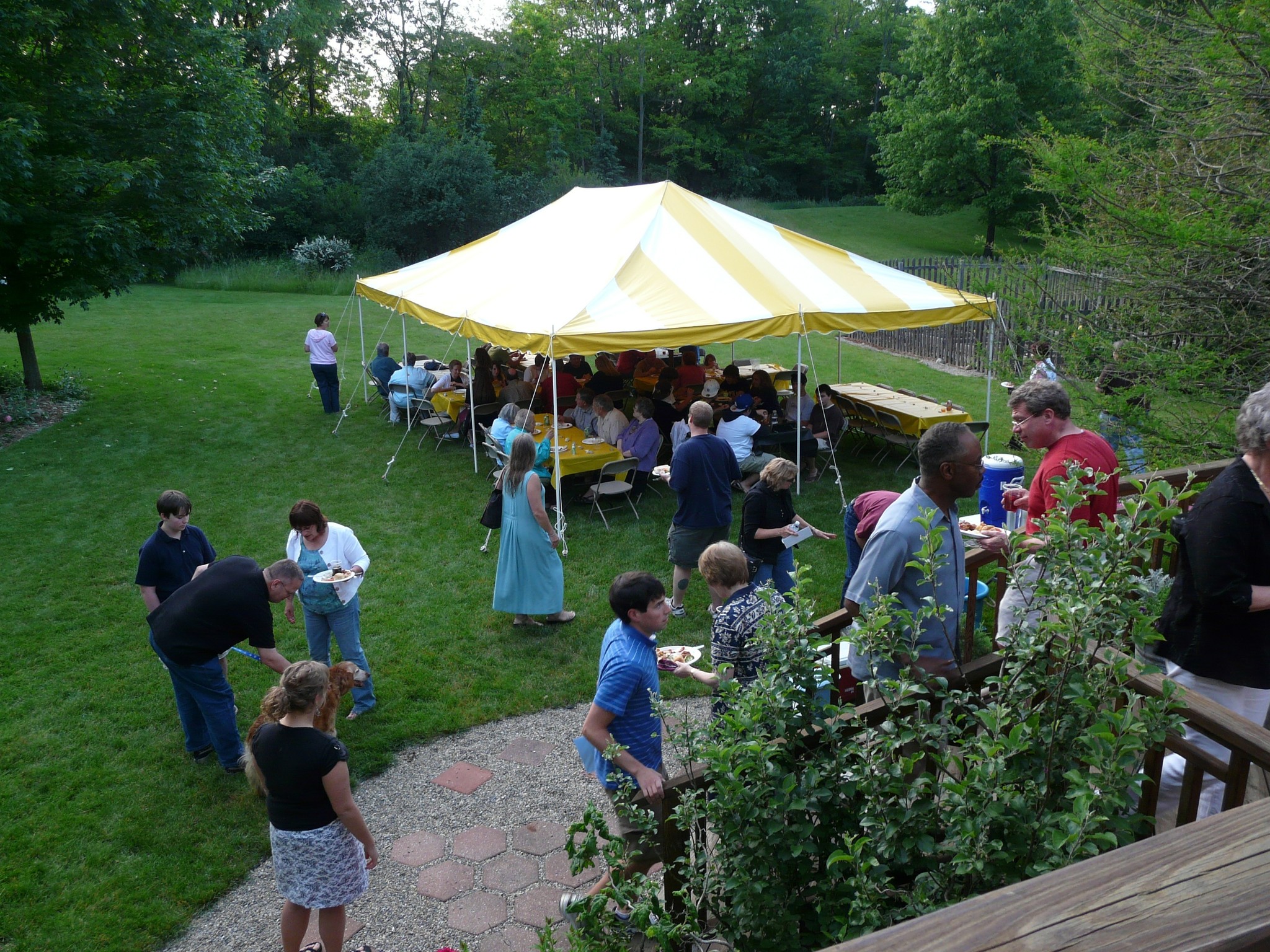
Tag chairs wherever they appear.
[359,345,808,527]
[813,382,990,482]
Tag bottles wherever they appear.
[782,521,800,544]
[764,414,769,424]
[771,410,778,428]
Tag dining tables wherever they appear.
[430,377,586,422]
[633,362,790,393]
[510,413,629,490]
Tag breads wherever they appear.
[976,523,1001,531]
[673,654,685,663]
[333,572,345,581]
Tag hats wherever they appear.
[702,379,719,398]
[730,394,753,412]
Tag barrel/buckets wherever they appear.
[963,577,996,631]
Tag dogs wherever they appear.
[236,659,373,800]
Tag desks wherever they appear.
[398,358,474,382]
[814,381,973,439]
[652,347,682,366]
[506,353,568,372]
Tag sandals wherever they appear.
[348,945,376,952]
[347,712,358,721]
[733,482,747,493]
[300,942,322,952]
[513,614,544,627]
[546,611,576,622]
[575,496,594,503]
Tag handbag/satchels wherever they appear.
[740,549,763,585]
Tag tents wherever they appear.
[355,181,996,538]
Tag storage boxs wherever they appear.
[818,642,859,704]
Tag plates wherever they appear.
[454,389,466,393]
[715,396,732,401]
[1001,382,1014,387]
[960,527,1011,539]
[576,379,585,382]
[706,368,718,372]
[462,395,466,398]
[532,429,541,435]
[705,373,720,377]
[718,370,723,375]
[551,423,572,429]
[655,646,702,668]
[313,569,355,583]
[550,446,567,452]
[652,466,670,476]
[651,374,659,378]
[582,438,605,444]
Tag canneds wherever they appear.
[543,415,550,426]
[584,427,591,438]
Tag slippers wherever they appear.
[805,476,817,483]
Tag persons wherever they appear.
[1002,341,1057,451]
[1095,340,1153,474]
[977,379,1120,650]
[304,312,346,414]
[673,541,793,748]
[559,571,671,940]
[370,344,903,613]
[134,490,239,714]
[145,558,305,774]
[251,660,379,952]
[844,421,986,796]
[284,499,376,721]
[1094,380,1270,822]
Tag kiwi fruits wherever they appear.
[684,655,694,662]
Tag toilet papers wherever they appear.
[756,409,768,416]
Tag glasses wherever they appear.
[785,478,795,483]
[1012,412,1036,428]
[947,459,984,470]
[281,582,294,600]
[320,312,325,318]
[295,523,312,533]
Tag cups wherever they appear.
[941,402,947,412]
[331,560,342,576]
[713,363,717,368]
[450,385,456,392]
[662,348,666,354]
[585,375,591,382]
[1003,484,1023,511]
[540,374,547,386]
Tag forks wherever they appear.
[652,477,661,481]
[963,538,980,547]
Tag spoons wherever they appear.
[970,544,982,548]
[665,661,677,667]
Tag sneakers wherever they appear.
[559,893,589,932]
[609,906,659,935]
[194,743,215,761]
[665,596,685,618]
[225,764,246,774]
[708,604,718,617]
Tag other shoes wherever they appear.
[469,444,474,448]
[443,433,459,438]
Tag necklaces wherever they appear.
[1250,468,1270,494]
